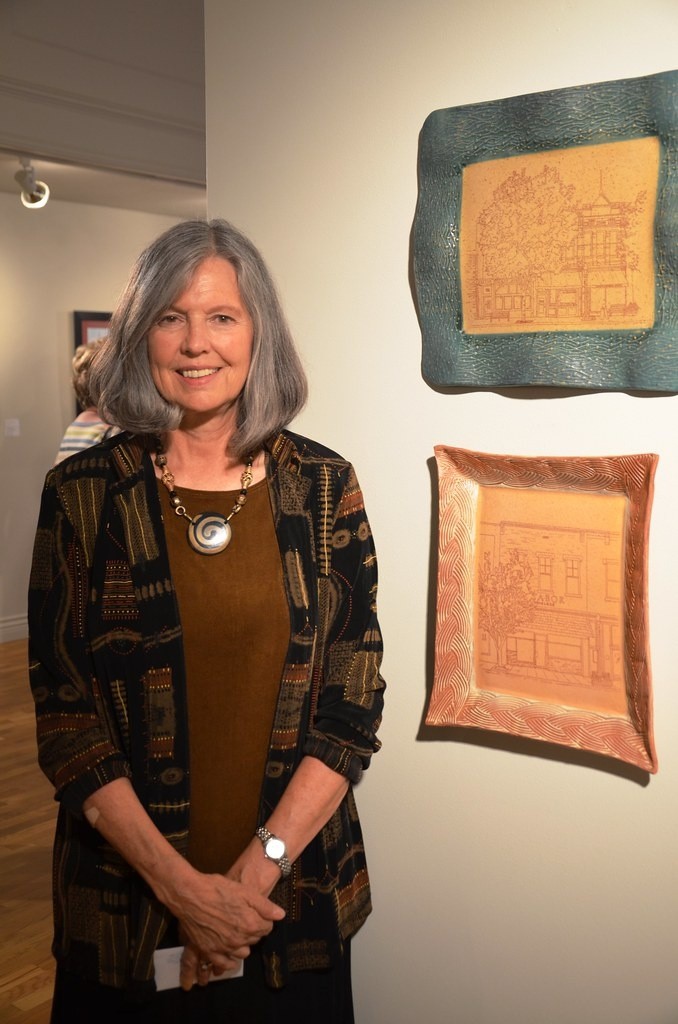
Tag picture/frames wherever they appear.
[73,310,112,416]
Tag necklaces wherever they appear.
[155,450,251,555]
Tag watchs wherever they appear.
[255,826,291,878]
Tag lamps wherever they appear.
[16,154,50,209]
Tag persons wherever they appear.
[53,335,120,466]
[28,206,387,1024]
